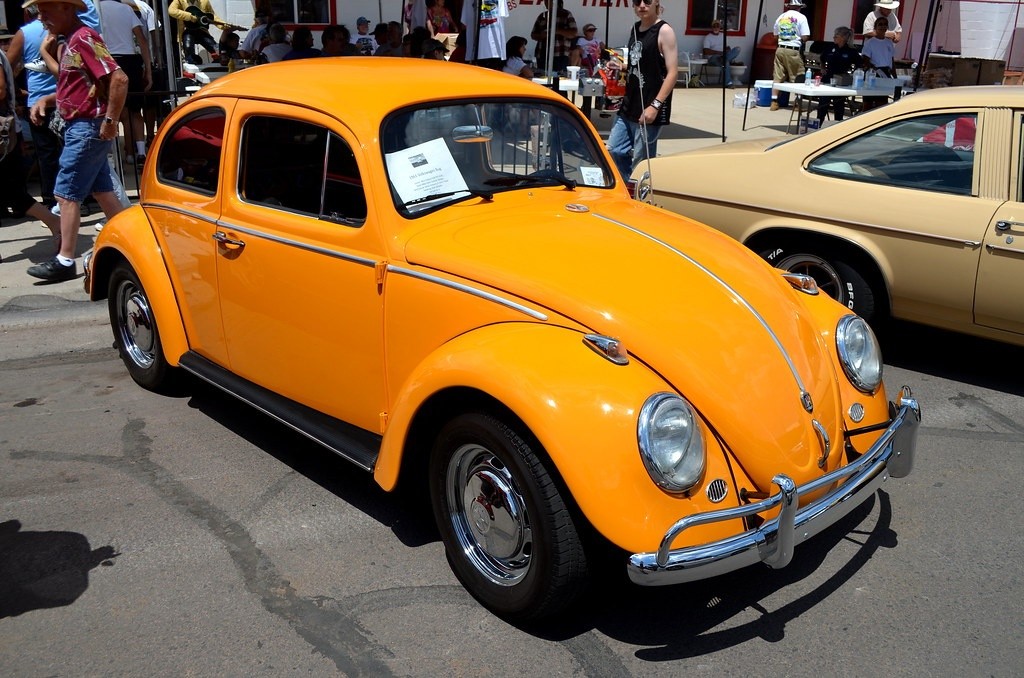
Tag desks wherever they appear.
[682,58,708,87]
[184,63,250,84]
[524,67,578,105]
[774,82,931,134]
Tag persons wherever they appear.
[605,0,679,182]
[530,0,578,98]
[20,0,129,281]
[96,1,153,169]
[216,7,320,60]
[6,0,58,211]
[370,21,449,61]
[702,20,741,90]
[119,0,162,163]
[348,16,378,55]
[122,2,156,162]
[317,25,361,55]
[503,36,533,79]
[569,25,610,72]
[817,0,907,128]
[404,0,459,37]
[769,0,809,111]
[167,0,231,64]
[0,48,62,263]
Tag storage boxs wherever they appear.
[732,97,756,108]
[754,80,790,107]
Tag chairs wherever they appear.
[699,52,724,86]
[660,48,691,89]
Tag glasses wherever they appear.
[713,25,720,28]
[632,0,652,6]
[588,29,596,32]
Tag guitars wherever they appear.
[182,5,250,32]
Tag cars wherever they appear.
[628,85,1024,347]
[84,56,922,626]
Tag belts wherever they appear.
[777,45,799,50]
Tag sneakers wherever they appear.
[23,57,51,73]
[27,256,76,281]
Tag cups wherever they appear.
[690,53,695,60]
[830,78,837,87]
[699,54,703,59]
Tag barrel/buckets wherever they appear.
[566,66,580,80]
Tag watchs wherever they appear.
[104,117,118,126]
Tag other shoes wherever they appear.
[770,102,779,111]
[54,233,61,254]
[717,56,724,62]
[795,104,807,111]
[94,217,109,231]
[727,84,734,88]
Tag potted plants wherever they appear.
[729,60,748,85]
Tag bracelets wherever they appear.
[619,63,622,71]
[650,99,663,110]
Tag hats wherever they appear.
[21,0,88,12]
[874,0,900,9]
[357,17,371,24]
[255,8,268,18]
[369,23,388,35]
[422,38,449,54]
[585,26,597,31]
[711,20,720,25]
[784,0,807,8]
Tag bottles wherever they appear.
[852,68,865,89]
[228,58,235,74]
[864,66,876,89]
[805,68,812,86]
[815,76,820,87]
